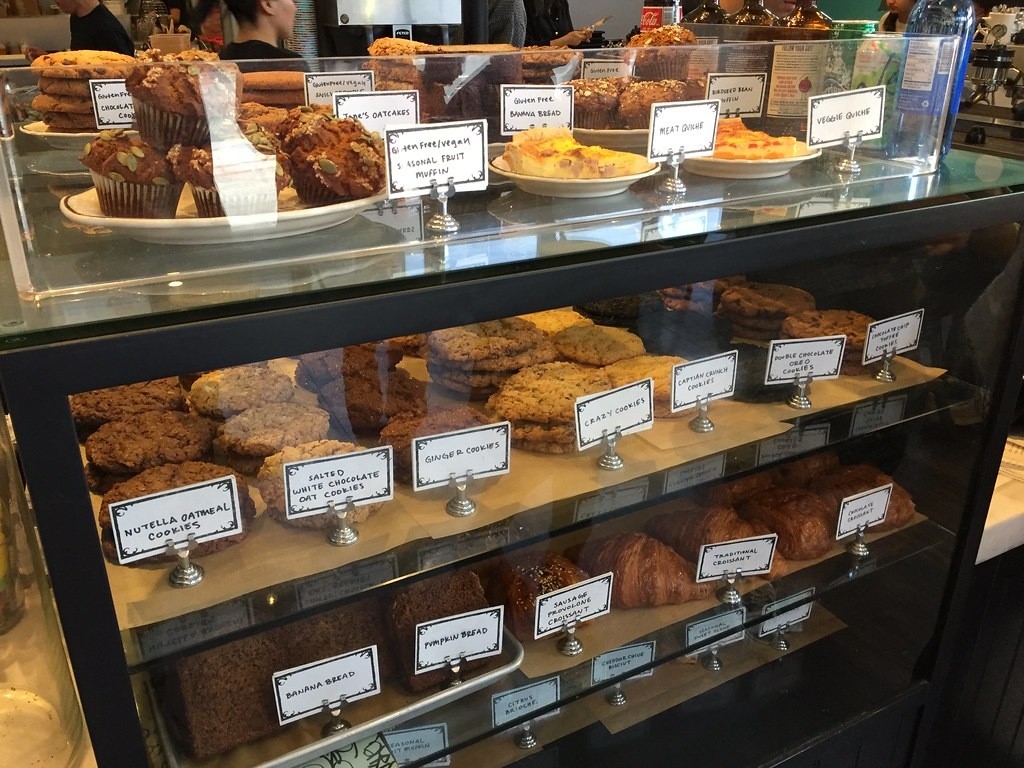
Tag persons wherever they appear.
[23,0,993,74]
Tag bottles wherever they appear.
[640,0,680,33]
[0,483,27,637]
[885,1,975,164]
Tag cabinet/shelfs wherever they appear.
[0,123,1024,768]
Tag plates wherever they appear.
[487,152,663,199]
[146,609,528,768]
[681,141,827,179]
[19,121,113,149]
[572,126,653,152]
[56,183,392,249]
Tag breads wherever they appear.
[30,22,916,760]
